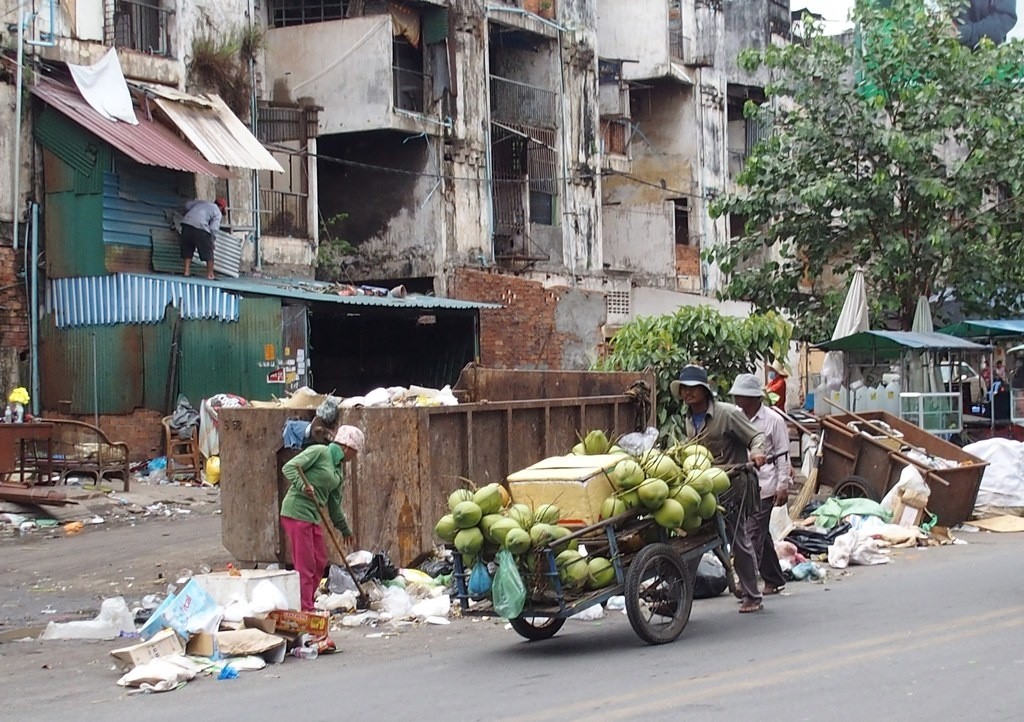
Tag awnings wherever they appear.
[808,329,995,429]
[0,49,287,180]
[932,319,1023,427]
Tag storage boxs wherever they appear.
[847,419,903,451]
[507,454,630,528]
[195,568,301,623]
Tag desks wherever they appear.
[0,423,55,485]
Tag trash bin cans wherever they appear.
[944,382,973,413]
[214,361,658,571]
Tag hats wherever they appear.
[670,364,717,399]
[334,425,365,453]
[727,373,766,397]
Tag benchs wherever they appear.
[20,418,129,492]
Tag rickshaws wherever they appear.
[444,449,788,644]
[771,406,991,528]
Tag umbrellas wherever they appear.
[903,296,949,435]
[819,265,871,412]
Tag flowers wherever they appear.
[10,387,30,404]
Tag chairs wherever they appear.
[162,415,202,482]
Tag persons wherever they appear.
[180,197,226,283]
[279,424,364,614]
[983,359,1006,390]
[767,360,786,413]
[725,373,795,596]
[671,365,766,613]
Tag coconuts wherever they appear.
[571,429,731,532]
[435,483,615,590]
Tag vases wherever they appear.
[14,403,23,423]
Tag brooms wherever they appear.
[788,428,825,523]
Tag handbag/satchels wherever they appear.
[468,556,494,602]
[493,551,526,619]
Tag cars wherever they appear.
[939,362,988,415]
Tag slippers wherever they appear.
[761,585,785,595]
[650,601,677,617]
[738,603,764,613]
